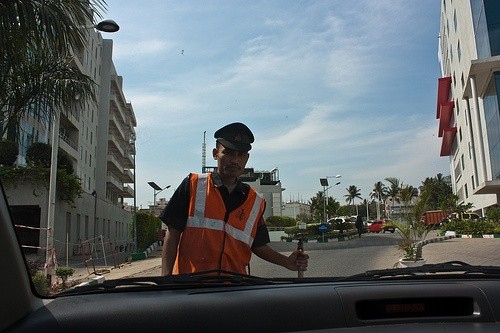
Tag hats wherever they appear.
[214,123,254,151]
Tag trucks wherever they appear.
[421,209,481,225]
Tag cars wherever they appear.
[328,215,395,234]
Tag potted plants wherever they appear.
[381,177,448,269]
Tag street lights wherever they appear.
[148,182,171,206]
[46,19,120,289]
[320,177,341,225]
